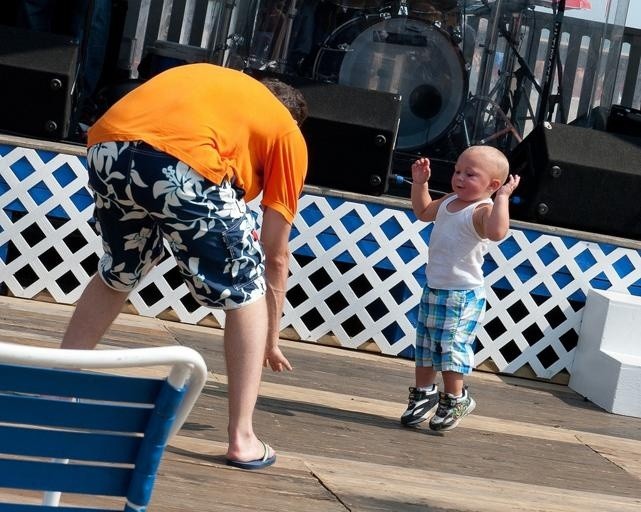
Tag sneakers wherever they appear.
[399,383,441,427]
[428,383,477,433]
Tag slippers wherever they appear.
[223,435,277,471]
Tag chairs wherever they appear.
[0,343,207,512]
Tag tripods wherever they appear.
[422,0,525,163]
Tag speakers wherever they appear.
[0,25,80,139]
[490,121,641,241]
[234,65,402,196]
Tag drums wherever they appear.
[310,13,468,150]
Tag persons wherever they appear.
[402,144,521,435]
[40,62,308,468]
